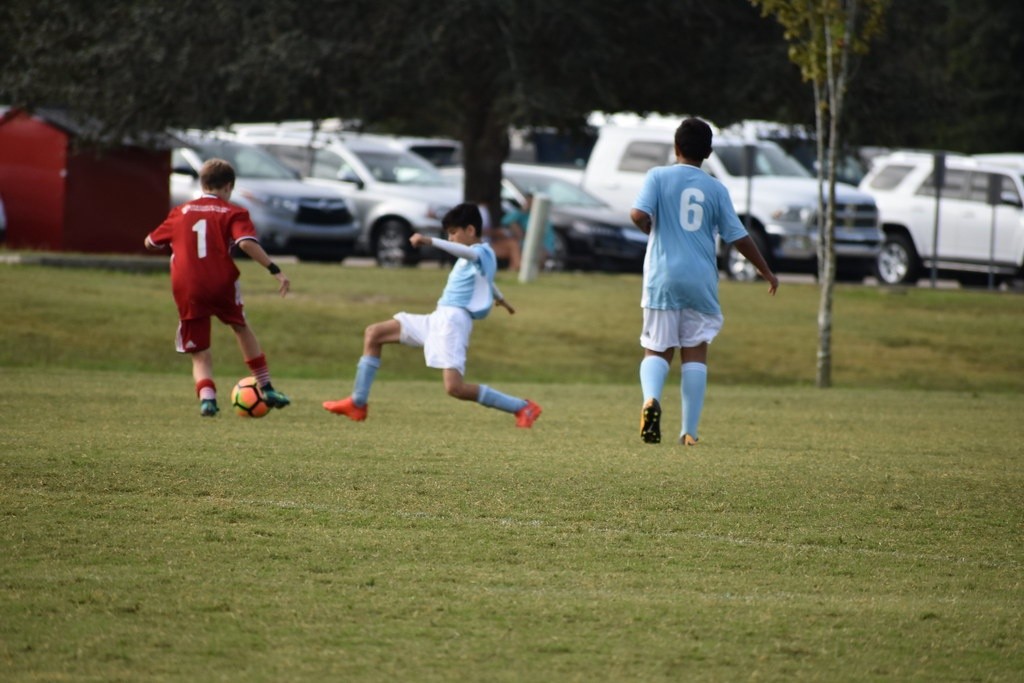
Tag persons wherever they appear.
[321,202,543,430]
[629,118,780,447]
[143,157,293,417]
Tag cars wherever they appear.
[0,80,1024,297]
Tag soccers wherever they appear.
[231,376,272,418]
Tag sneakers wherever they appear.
[262,391,290,409]
[322,397,367,422]
[202,398,216,416]
[515,399,541,428]
[639,399,662,444]
[681,434,702,446]
